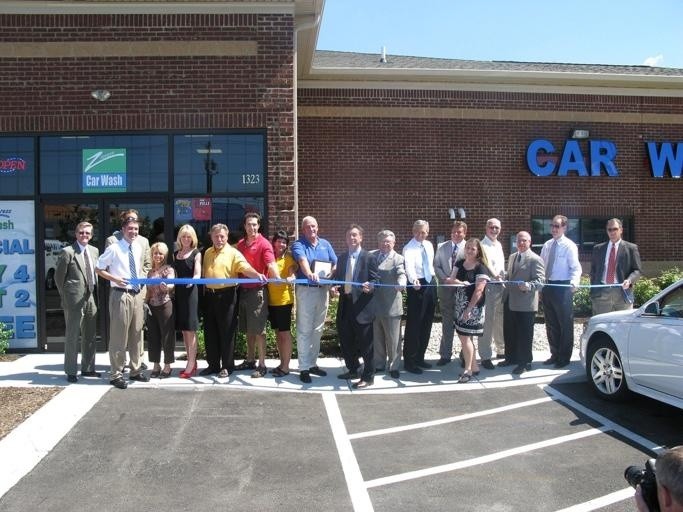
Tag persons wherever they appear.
[402,220,434,375]
[479,218,506,370]
[329,223,378,389]
[95,216,149,389]
[171,225,201,379]
[235,212,282,379]
[367,230,407,378]
[635,446,683,512]
[145,242,175,379]
[290,216,337,383]
[434,220,468,368]
[104,209,152,373]
[54,222,100,383]
[266,230,299,377]
[498,231,544,370]
[199,224,267,377]
[588,218,642,317]
[537,215,582,368]
[449,238,491,383]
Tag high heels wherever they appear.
[150,368,161,378]
[159,368,172,379]
[180,364,197,378]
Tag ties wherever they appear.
[345,256,353,294]
[606,243,615,285]
[546,242,557,281]
[378,254,385,264]
[129,244,139,293]
[453,244,458,268]
[84,249,94,293]
[421,243,432,284]
[518,254,522,265]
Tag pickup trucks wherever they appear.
[44,239,64,289]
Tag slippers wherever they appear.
[458,373,472,383]
[272,370,289,377]
[269,367,281,373]
[472,370,481,375]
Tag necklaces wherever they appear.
[177,247,189,258]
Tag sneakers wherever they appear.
[234,361,256,370]
[251,366,267,378]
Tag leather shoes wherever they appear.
[309,367,327,376]
[389,370,400,378]
[130,373,150,382]
[199,367,220,375]
[82,371,100,378]
[554,359,571,368]
[497,359,514,365]
[436,358,451,365]
[544,357,559,365]
[68,374,78,383]
[513,363,532,374]
[407,366,423,374]
[300,372,311,383]
[481,359,494,369]
[418,362,431,368]
[110,378,128,390]
[338,372,362,379]
[220,366,233,377]
[353,380,374,388]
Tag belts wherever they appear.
[546,280,570,284]
[299,283,328,287]
[205,286,236,294]
[114,284,144,293]
[603,286,620,288]
[345,294,351,299]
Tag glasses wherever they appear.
[550,223,563,228]
[607,227,620,232]
[125,217,141,222]
[78,231,91,235]
[379,240,393,244]
[487,226,500,229]
[516,239,528,242]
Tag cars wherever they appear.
[578,278,682,418]
